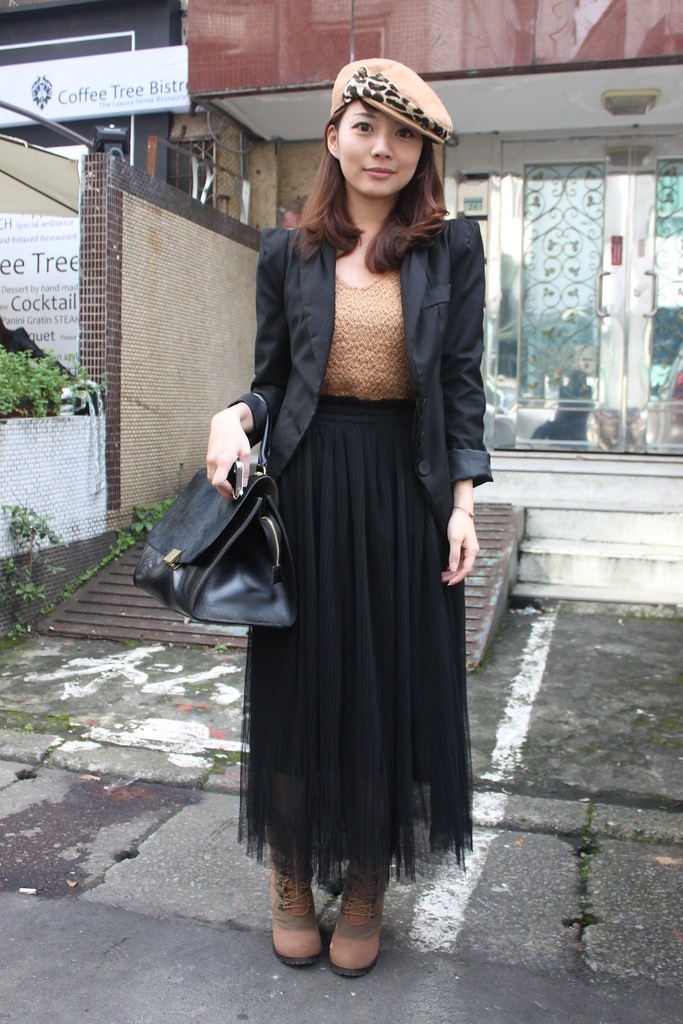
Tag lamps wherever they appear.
[607,146,653,168]
[601,88,661,117]
[92,124,130,156]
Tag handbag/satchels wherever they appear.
[132,390,298,627]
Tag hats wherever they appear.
[330,58,453,144]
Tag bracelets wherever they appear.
[453,505,474,517]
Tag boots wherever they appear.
[268,768,323,965]
[328,779,393,976]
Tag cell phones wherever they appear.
[228,462,245,497]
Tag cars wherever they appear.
[648,342,683,451]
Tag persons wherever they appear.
[205,59,523,975]
